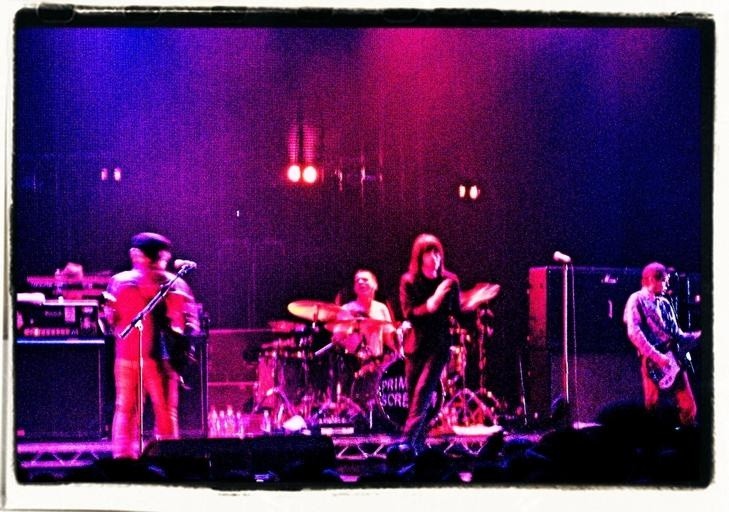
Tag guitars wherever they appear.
[647,326,704,391]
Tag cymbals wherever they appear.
[287,299,349,322]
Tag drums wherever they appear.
[352,359,444,429]
[254,350,327,410]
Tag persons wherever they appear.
[102,233,201,458]
[623,263,698,424]
[330,270,395,373]
[400,234,478,451]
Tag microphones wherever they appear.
[174,258,198,270]
[553,251,571,262]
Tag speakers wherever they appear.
[144,441,236,482]
[249,434,339,484]
